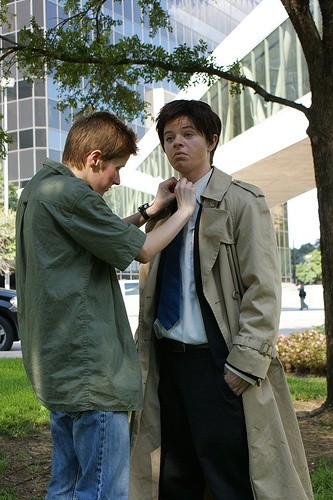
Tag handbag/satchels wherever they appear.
[299,289,306,297]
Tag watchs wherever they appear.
[137,203,151,221]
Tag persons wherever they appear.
[15,111,197,500]
[299,283,308,310]
[128,100,314,500]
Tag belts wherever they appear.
[156,336,210,353]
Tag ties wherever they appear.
[155,198,184,331]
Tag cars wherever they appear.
[0,288,21,350]
